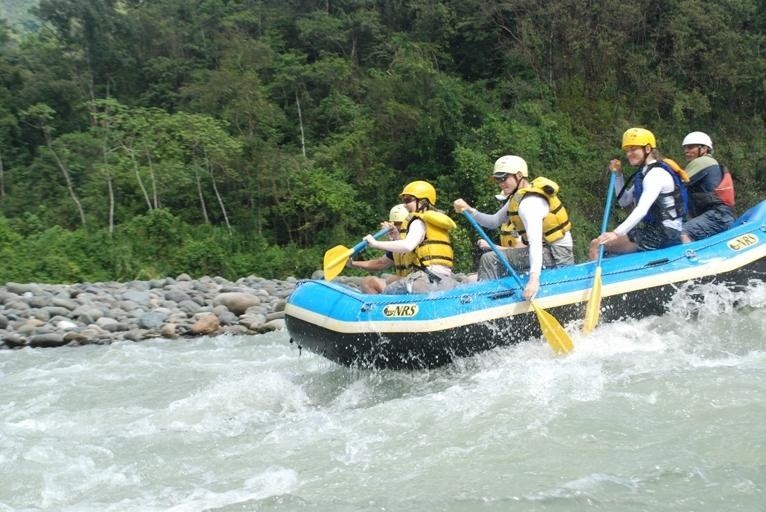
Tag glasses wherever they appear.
[495,177,507,183]
[403,196,416,203]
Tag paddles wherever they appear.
[583,160,618,334]
[322,227,396,282]
[460,208,574,355]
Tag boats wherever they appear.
[285,200,766,368]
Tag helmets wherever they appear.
[400,180,436,206]
[621,128,656,149]
[388,203,409,221]
[494,155,529,177]
[683,132,713,151]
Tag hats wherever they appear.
[490,172,506,178]
[494,194,506,201]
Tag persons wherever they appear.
[665,132,734,246]
[588,128,684,261]
[453,154,573,279]
[345,179,455,295]
